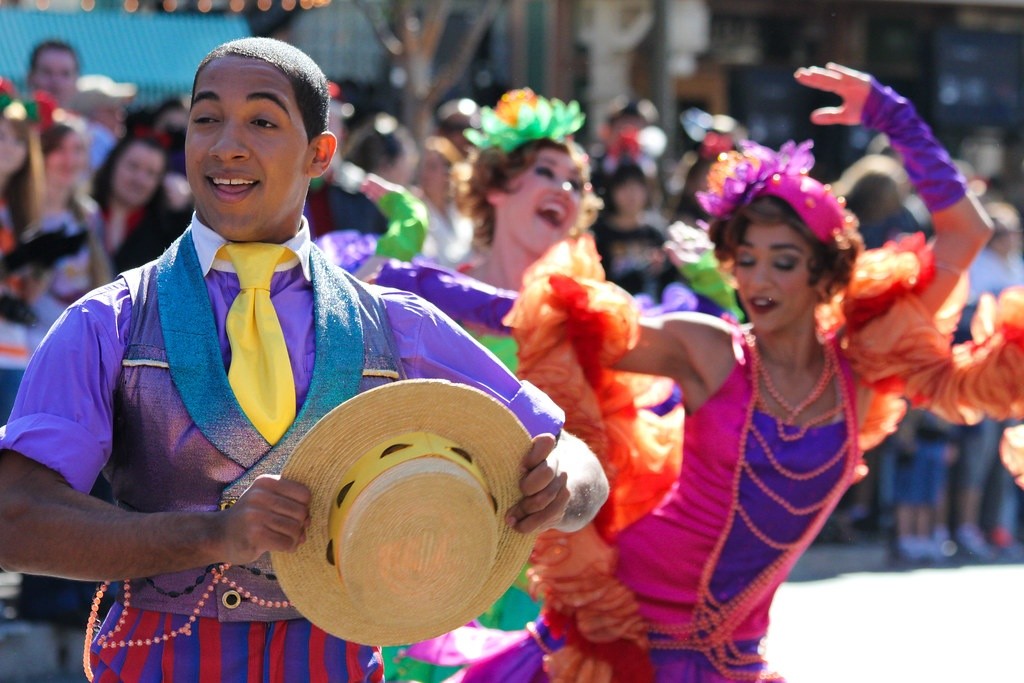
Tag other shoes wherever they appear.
[903,523,1024,562]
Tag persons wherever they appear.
[383,88,593,683]
[0,40,1024,567]
[0,36,610,683]
[369,62,996,683]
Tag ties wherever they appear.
[217,242,301,449]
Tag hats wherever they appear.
[69,75,136,112]
[270,378,547,647]
